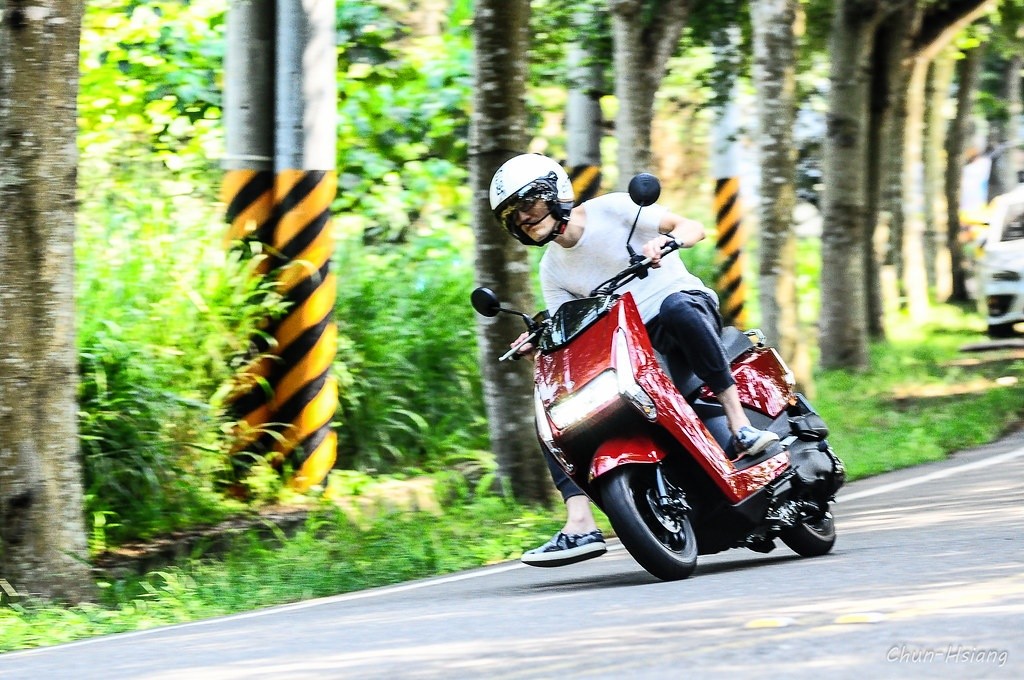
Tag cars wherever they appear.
[983,190,1023,337]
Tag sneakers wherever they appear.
[726,426,779,462]
[521,529,607,567]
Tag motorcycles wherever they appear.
[473,173,848,583]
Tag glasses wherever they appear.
[505,190,539,225]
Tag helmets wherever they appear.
[489,153,573,247]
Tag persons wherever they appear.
[489,152,780,568]
